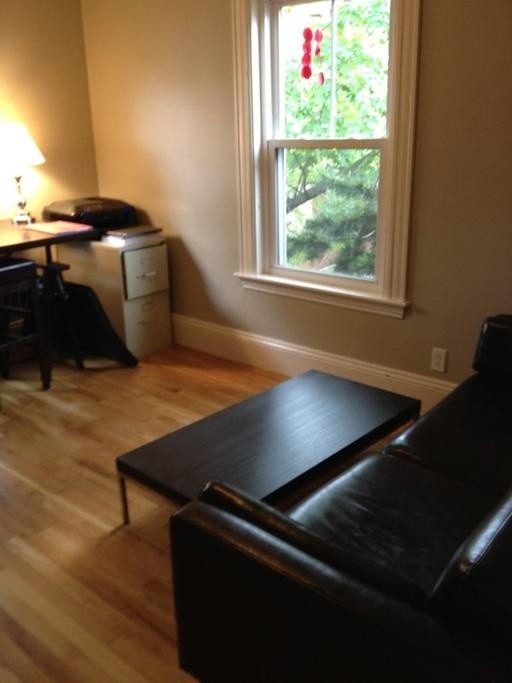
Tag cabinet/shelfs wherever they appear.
[57,235,174,359]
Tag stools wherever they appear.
[1,255,57,391]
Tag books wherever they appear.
[20,219,95,238]
[100,225,167,248]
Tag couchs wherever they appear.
[168,307,510,681]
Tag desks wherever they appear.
[0,216,97,372]
